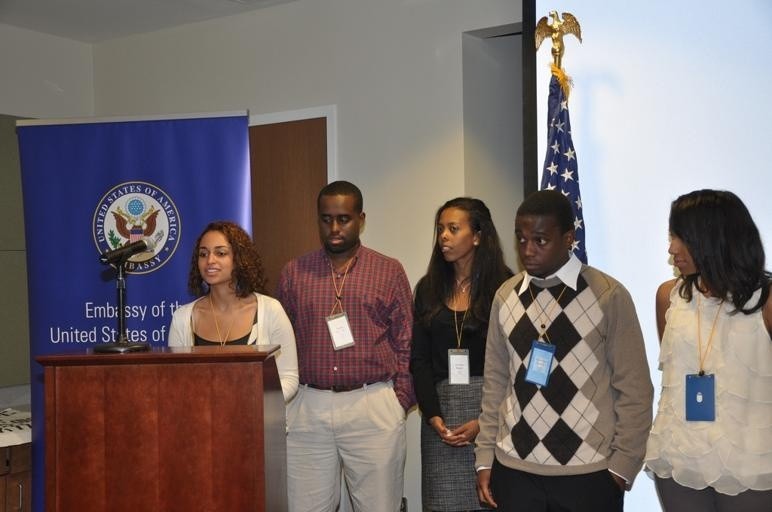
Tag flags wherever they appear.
[538,67,589,267]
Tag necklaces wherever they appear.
[454,278,472,293]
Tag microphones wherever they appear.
[98,237,153,265]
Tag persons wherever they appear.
[166,219,301,404]
[639,188,770,511]
[279,181,418,510]
[408,195,517,510]
[472,190,653,512]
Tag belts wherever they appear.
[300,381,378,392]
